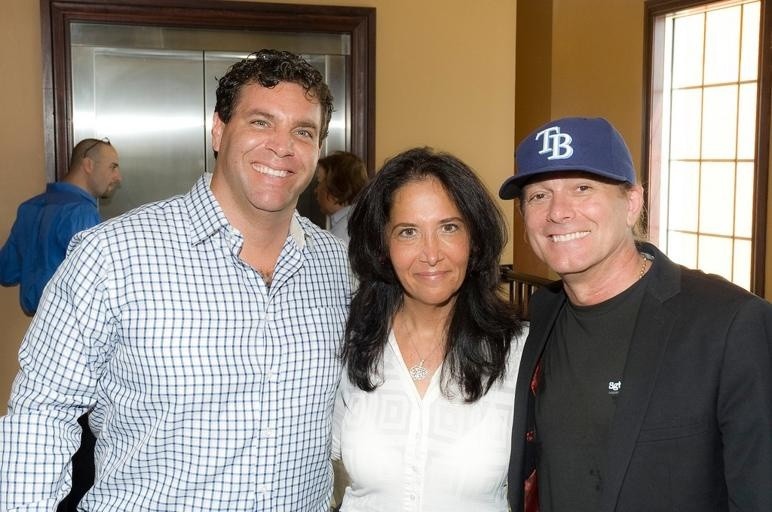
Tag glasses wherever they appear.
[84,137,110,157]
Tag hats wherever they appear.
[499,118,638,199]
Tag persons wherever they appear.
[1,138,121,512]
[326,145,530,512]
[499,114,770,512]
[312,152,371,240]
[0,48,361,512]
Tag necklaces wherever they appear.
[638,252,648,279]
[399,307,449,383]
